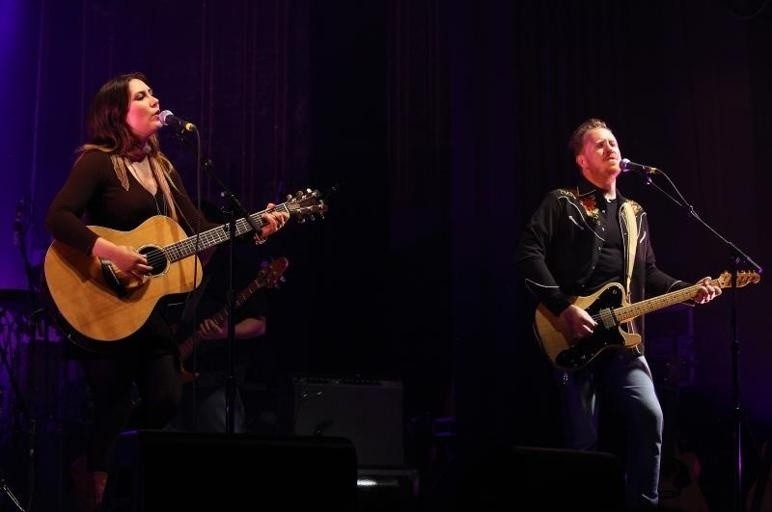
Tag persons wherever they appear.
[140,197,268,434]
[44,72,293,512]
[515,116,725,512]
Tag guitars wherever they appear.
[131,255,288,410]
[534,267,760,372]
[45,188,330,350]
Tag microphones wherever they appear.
[159,109,193,133]
[619,158,657,175]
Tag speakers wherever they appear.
[434,431,620,512]
[102,429,358,512]
[293,378,404,465]
